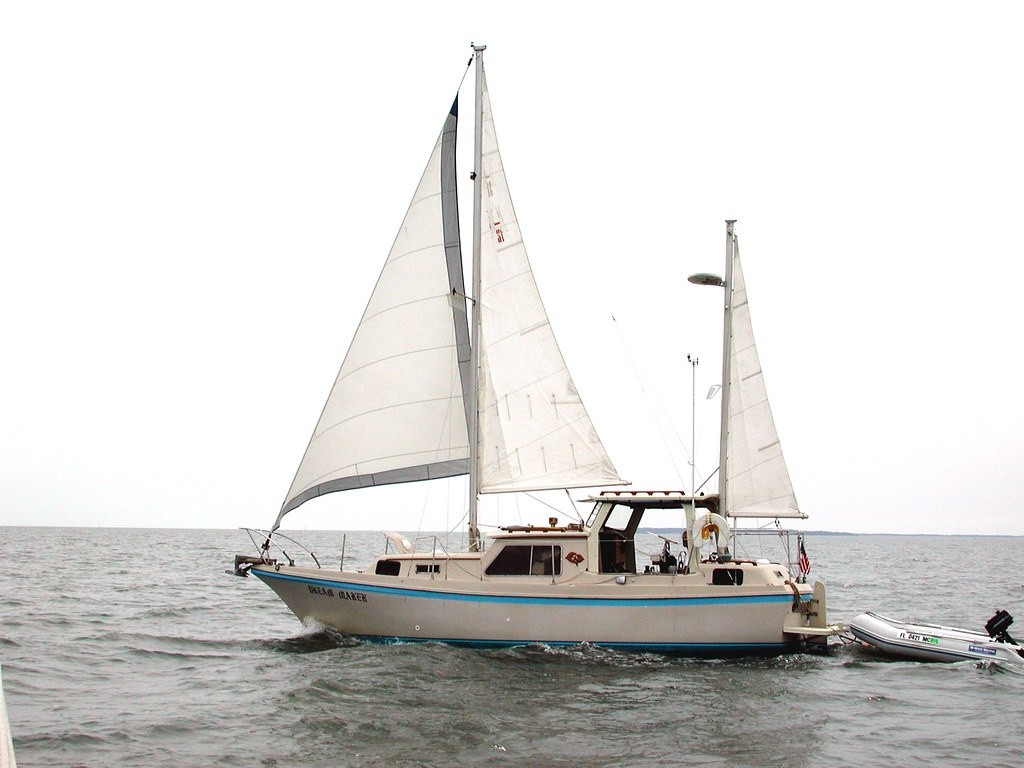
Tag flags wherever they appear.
[798,542,811,575]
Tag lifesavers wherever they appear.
[691,513,731,549]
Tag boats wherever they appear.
[850,610,1024,667]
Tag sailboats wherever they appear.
[225,42,849,657]
